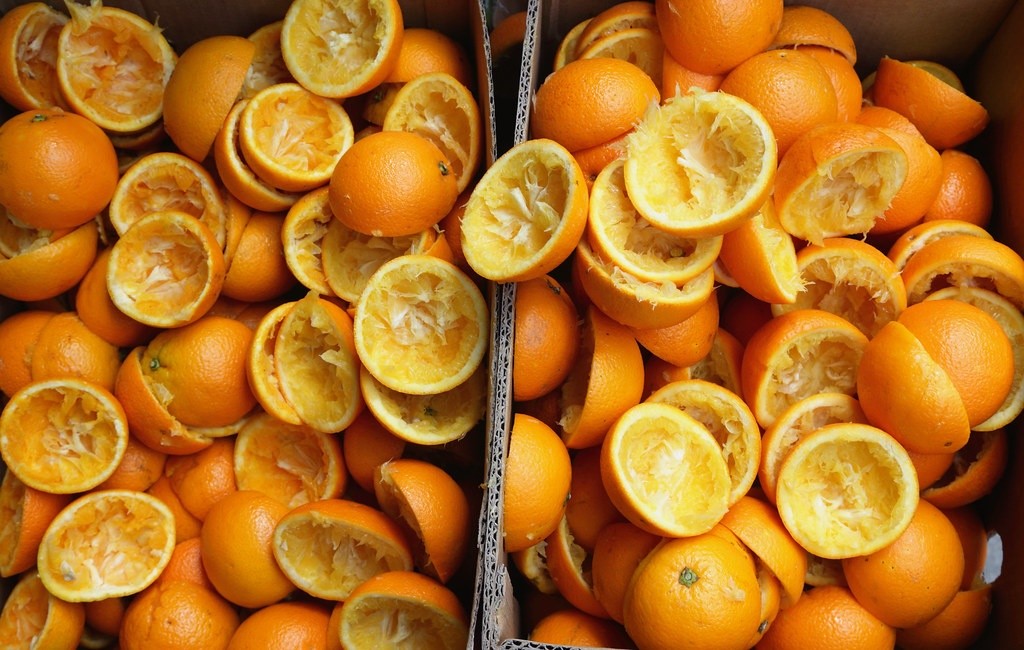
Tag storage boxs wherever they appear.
[0,0,1024,650]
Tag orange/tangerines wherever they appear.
[0,110,117,232]
